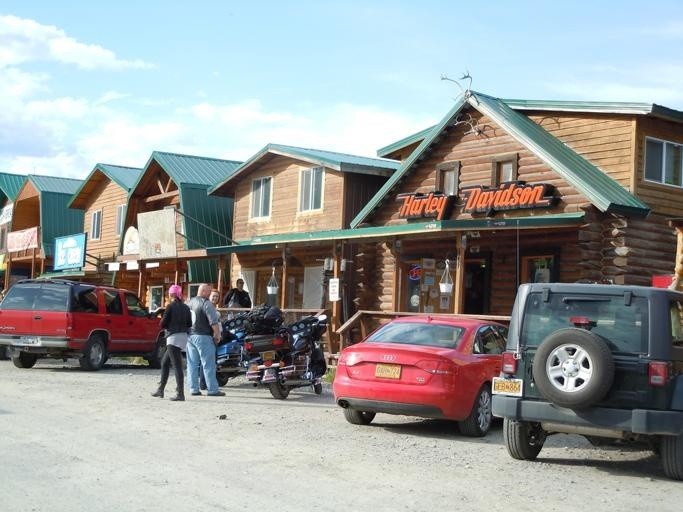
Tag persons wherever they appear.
[187,283,225,396]
[200,289,220,391]
[152,286,192,401]
[223,280,251,314]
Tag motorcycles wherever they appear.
[245,315,326,399]
[216,332,247,386]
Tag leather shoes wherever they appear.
[151,389,165,398]
[170,392,185,401]
[207,391,226,396]
[192,391,202,396]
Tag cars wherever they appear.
[334,314,509,438]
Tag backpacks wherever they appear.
[241,302,286,334]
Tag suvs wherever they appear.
[491,282,682,481]
[0,278,166,371]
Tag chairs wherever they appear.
[598,307,642,351]
